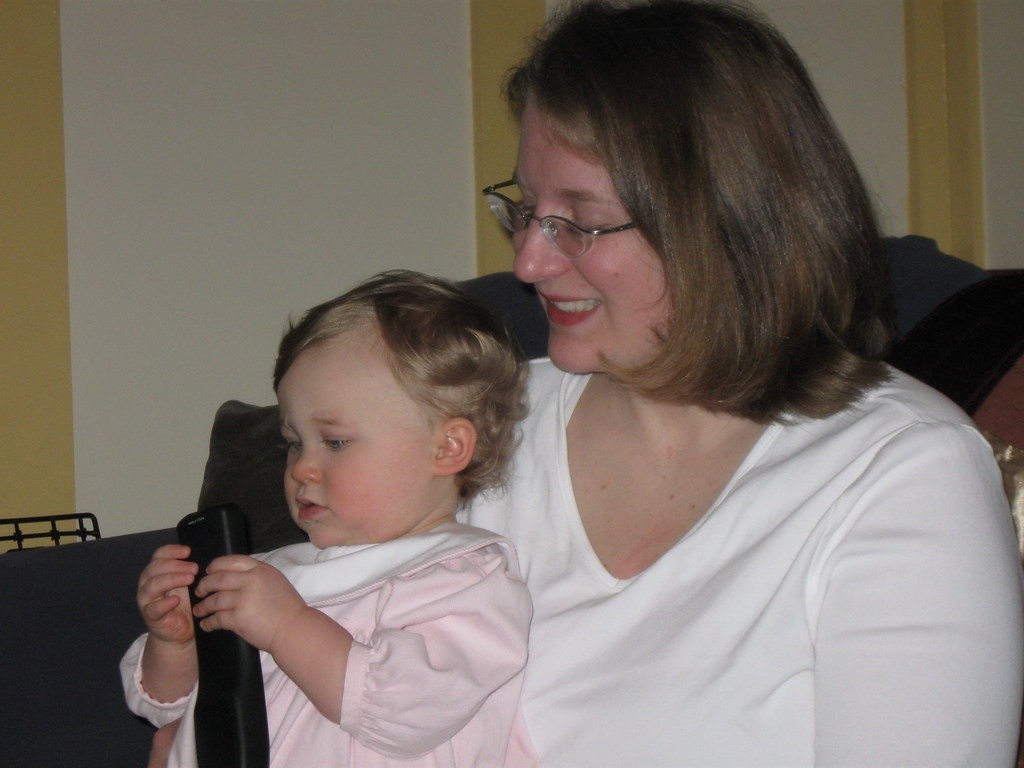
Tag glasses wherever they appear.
[483,180,641,259]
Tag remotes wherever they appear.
[176,502,270,768]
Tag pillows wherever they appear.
[201,397,311,557]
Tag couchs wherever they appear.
[0,228,1024,768]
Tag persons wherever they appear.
[119,269,535,768]
[149,0,1023,768]
